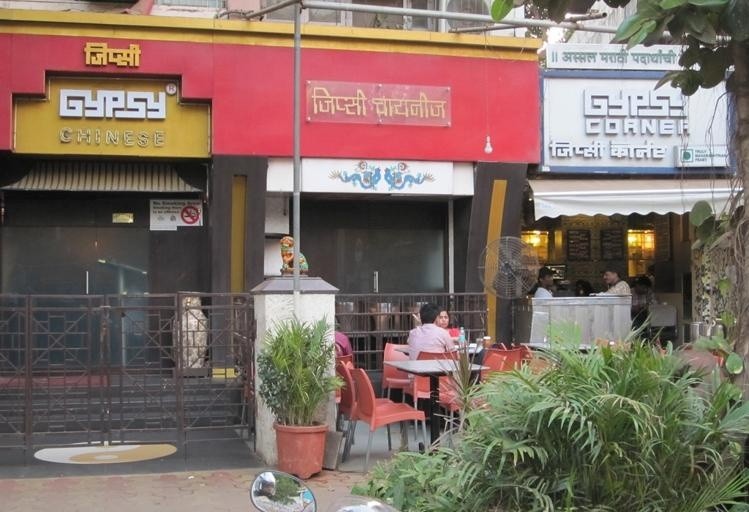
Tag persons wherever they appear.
[407,302,459,391]
[533,266,655,328]
[412,305,461,337]
[335,329,353,356]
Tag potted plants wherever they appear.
[257,309,351,481]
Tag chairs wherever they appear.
[336,343,534,477]
[647,304,682,348]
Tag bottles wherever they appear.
[458,326,466,346]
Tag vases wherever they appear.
[172,296,207,369]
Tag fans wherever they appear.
[478,236,540,343]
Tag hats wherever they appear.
[540,267,556,278]
[600,265,621,274]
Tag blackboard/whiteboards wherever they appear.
[568,231,591,261]
[601,229,624,261]
